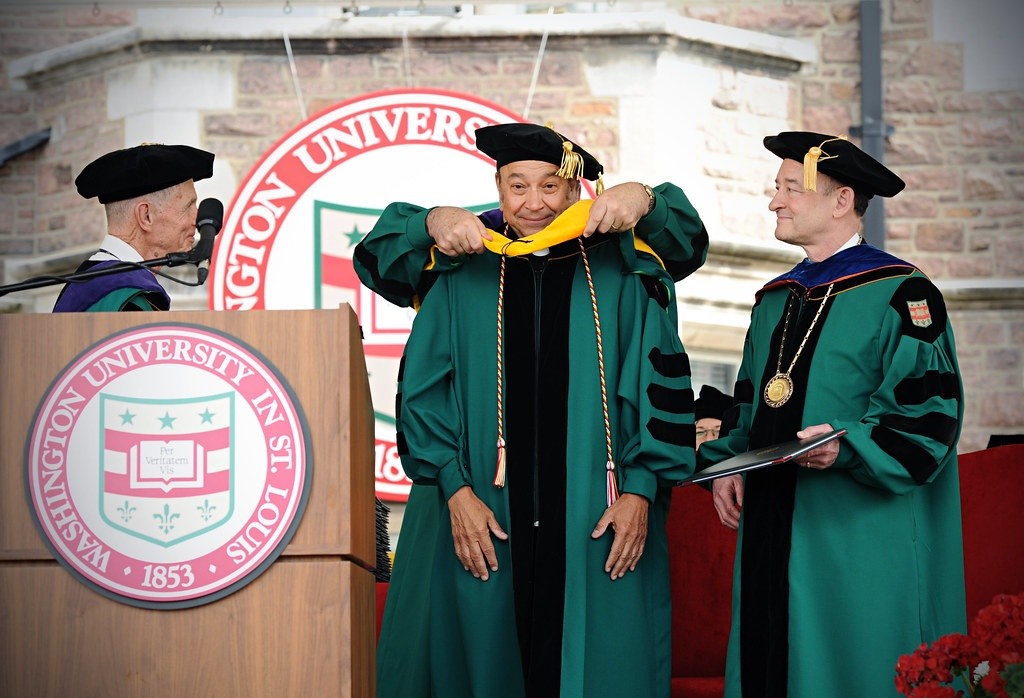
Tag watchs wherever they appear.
[641,181,655,213]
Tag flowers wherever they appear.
[892,592,1024,698]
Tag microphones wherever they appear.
[196,198,224,285]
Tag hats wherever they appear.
[762,131,905,200]
[474,123,605,180]
[74,145,215,205]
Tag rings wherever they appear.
[611,225,619,231]
[807,461,811,468]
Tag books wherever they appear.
[676,427,847,487]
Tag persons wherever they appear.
[51,143,216,312]
[353,120,710,698]
[693,385,735,452]
[697,131,971,698]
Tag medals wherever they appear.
[762,373,793,409]
